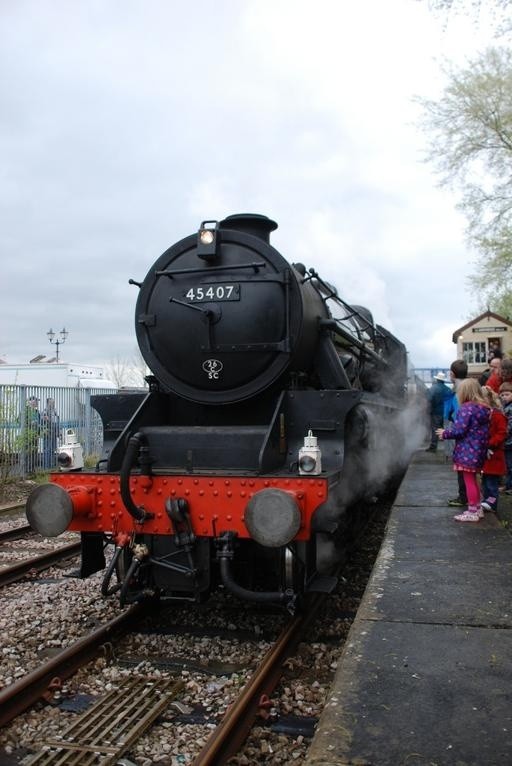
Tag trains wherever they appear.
[23,212,431,628]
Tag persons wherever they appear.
[39,397,62,471]
[424,355,512,524]
[16,397,50,477]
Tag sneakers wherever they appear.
[454,508,484,522]
[481,498,497,512]
[426,448,437,452]
[447,496,465,505]
[506,489,512,495]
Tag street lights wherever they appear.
[46,326,71,362]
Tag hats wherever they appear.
[434,374,447,382]
[499,382,512,392]
